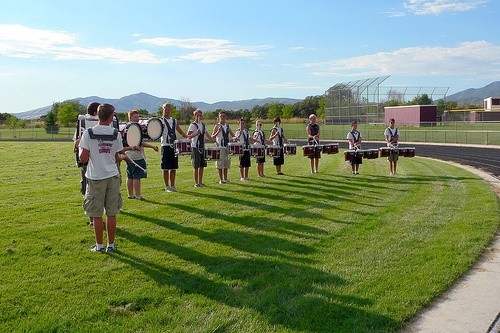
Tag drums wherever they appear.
[251,145,265,158]
[267,144,281,157]
[344,147,415,161]
[205,146,228,160]
[138,116,164,141]
[118,121,143,148]
[173,139,193,156]
[302,143,339,157]
[227,142,244,156]
[283,143,297,155]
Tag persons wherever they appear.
[251,119,266,176]
[212,110,238,184]
[71,102,103,227]
[122,109,159,200]
[188,108,216,187]
[158,102,188,192]
[306,113,320,173]
[346,120,361,174]
[268,117,287,174]
[76,102,125,253]
[235,116,257,181]
[383,118,400,175]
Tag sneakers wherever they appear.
[258,175,266,178]
[276,172,284,175]
[136,195,147,200]
[194,183,207,188]
[165,186,179,192]
[90,243,116,252]
[352,171,359,174]
[88,222,93,226]
[240,177,251,181]
[311,170,319,174]
[128,196,136,199]
[219,180,230,184]
[390,172,397,176]
